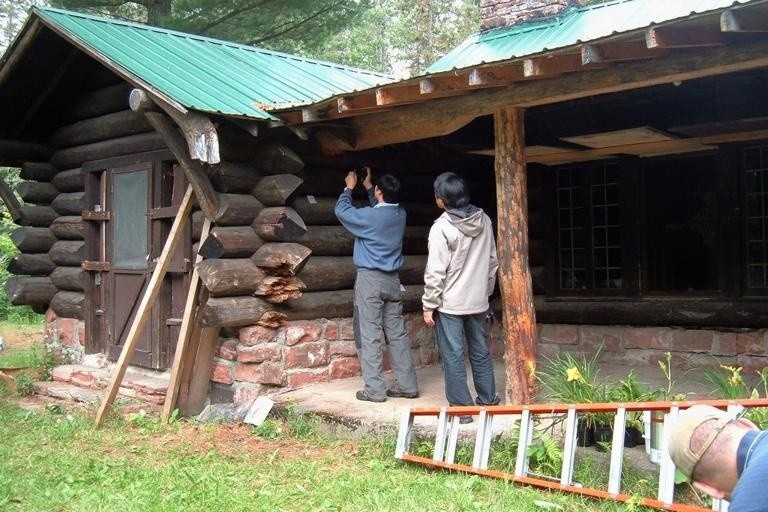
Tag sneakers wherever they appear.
[386,380,420,399]
[355,388,388,402]
[436,408,474,424]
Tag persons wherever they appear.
[421,171,499,424]
[335,167,419,402]
[669,400,767,512]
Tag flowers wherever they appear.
[524,338,768,435]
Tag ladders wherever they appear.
[394,397,768,512]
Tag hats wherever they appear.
[664,402,733,483]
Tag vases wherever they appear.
[576,420,645,454]
